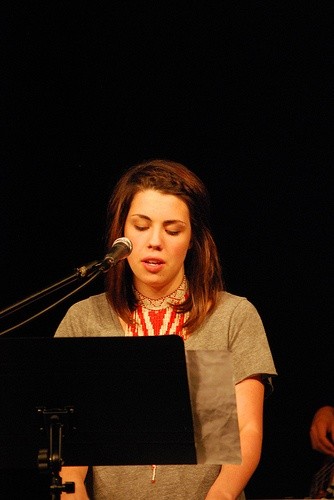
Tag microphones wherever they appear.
[98,235,133,275]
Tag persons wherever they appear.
[51,157,275,500]
